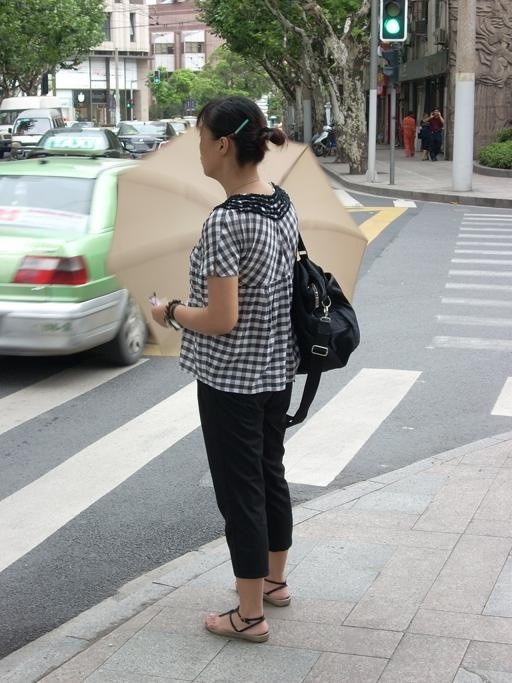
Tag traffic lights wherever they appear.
[127,96,131,108]
[381,48,399,83]
[379,0,408,42]
[153,69,160,84]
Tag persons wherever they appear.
[422,107,444,161]
[420,112,432,161]
[402,111,416,158]
[152,97,302,643]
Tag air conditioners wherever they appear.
[430,26,448,46]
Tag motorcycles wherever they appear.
[311,120,338,157]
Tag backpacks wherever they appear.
[284,231,361,427]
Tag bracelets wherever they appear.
[163,299,184,329]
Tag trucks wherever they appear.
[1,95,68,158]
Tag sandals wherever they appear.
[236,576,291,607]
[205,605,270,645]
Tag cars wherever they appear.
[26,126,135,159]
[64,115,198,138]
[0,154,148,365]
[267,120,283,131]
[116,120,183,160]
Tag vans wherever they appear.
[8,108,65,159]
